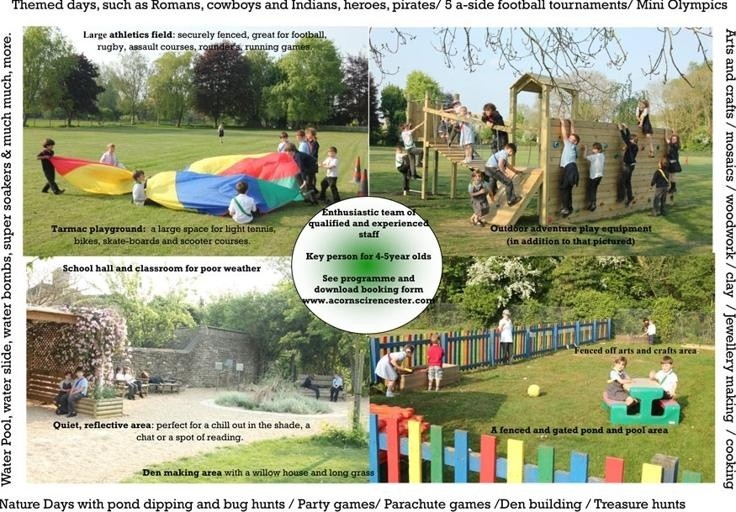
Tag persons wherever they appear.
[141,371,177,384]
[376,344,414,398]
[607,356,638,408]
[582,141,605,211]
[400,121,425,178]
[395,143,411,196]
[55,371,71,414]
[123,366,146,398]
[330,371,343,402]
[558,117,579,217]
[228,181,259,224]
[98,143,125,172]
[439,100,508,164]
[663,127,681,195]
[614,122,639,207]
[469,170,495,227]
[427,334,444,392]
[115,366,136,400]
[649,158,670,218]
[650,356,678,414]
[498,309,513,366]
[131,169,163,208]
[486,143,523,207]
[35,137,66,195]
[641,317,656,345]
[67,365,87,417]
[216,123,226,144]
[636,99,655,159]
[302,372,320,401]
[276,127,342,206]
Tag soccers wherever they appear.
[528,384,540,398]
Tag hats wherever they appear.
[502,310,510,315]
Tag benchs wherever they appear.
[660,398,682,427]
[601,386,632,427]
[297,374,347,401]
[115,375,181,398]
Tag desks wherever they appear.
[601,376,682,427]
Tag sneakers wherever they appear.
[474,219,484,227]
[508,197,522,206]
[386,391,395,397]
